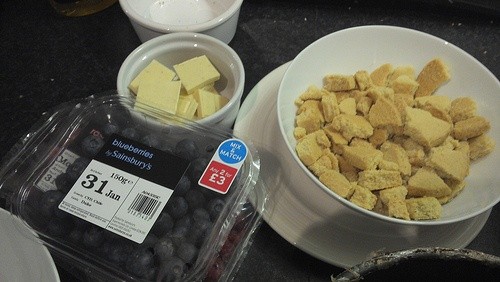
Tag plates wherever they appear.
[231,60,492,269]
[0,207,62,282]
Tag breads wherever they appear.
[294,58,492,220]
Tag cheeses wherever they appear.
[128,54,230,122]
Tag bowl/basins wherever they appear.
[277,24,500,226]
[331,248,500,282]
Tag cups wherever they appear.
[116,32,246,150]
[119,0,243,47]
